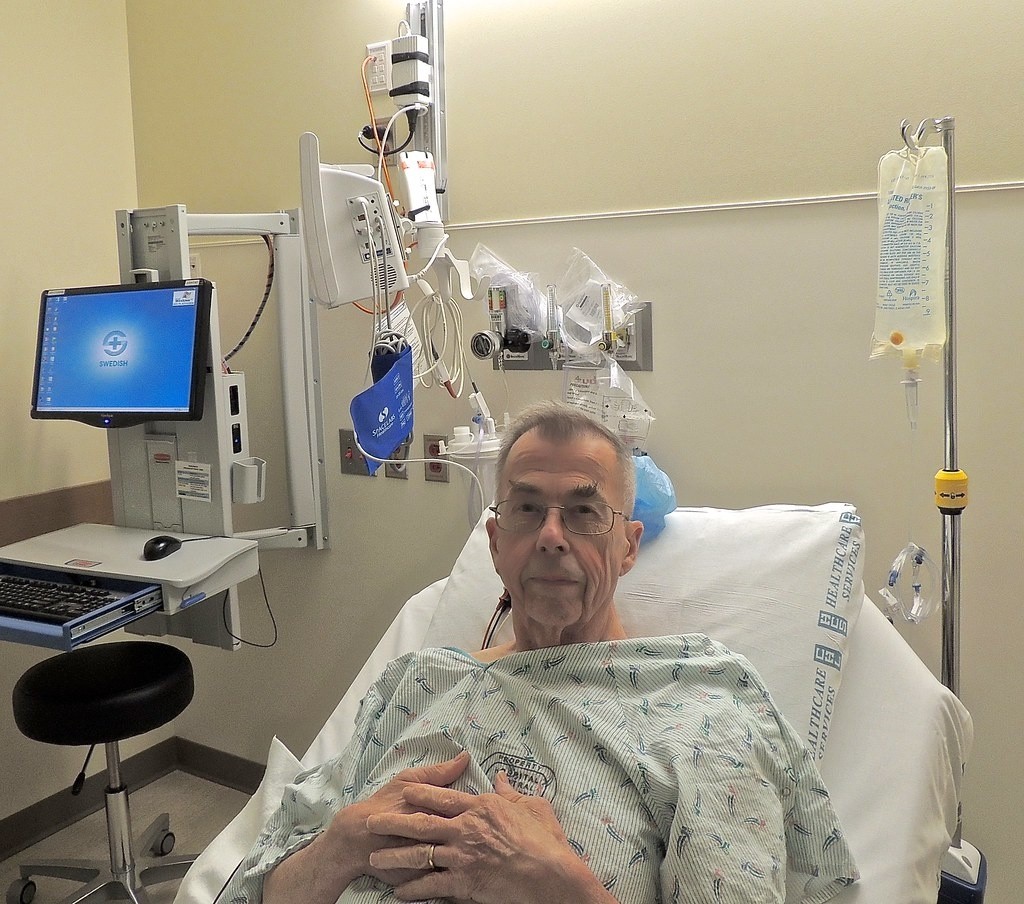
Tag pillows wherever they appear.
[420,503,864,774]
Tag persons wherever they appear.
[250,397,791,904]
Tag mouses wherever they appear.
[143,535,182,560]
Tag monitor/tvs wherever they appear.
[31,278,212,429]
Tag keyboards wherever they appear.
[0,575,121,626]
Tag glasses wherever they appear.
[489,498,629,535]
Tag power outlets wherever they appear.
[385,438,409,479]
[423,435,450,484]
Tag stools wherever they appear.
[6,642,201,904]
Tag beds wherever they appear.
[173,575,988,904]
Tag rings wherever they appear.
[428,844,437,868]
